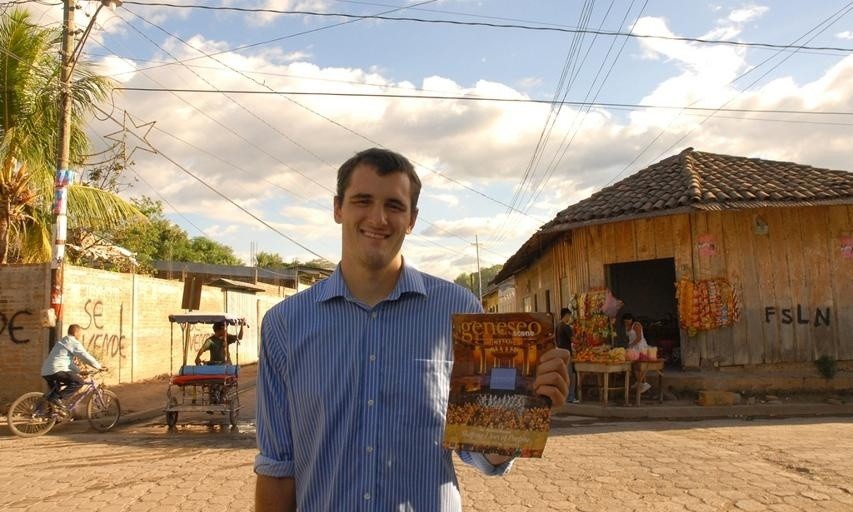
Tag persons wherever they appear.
[622,312,652,395]
[40,324,108,426]
[253,146,572,512]
[195,318,245,433]
[555,308,580,404]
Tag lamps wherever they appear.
[563,233,572,248]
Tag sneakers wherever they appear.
[631,381,651,394]
[567,399,580,404]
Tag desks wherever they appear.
[571,357,666,406]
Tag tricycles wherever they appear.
[163,315,246,426]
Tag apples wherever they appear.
[591,351,624,364]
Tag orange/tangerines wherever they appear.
[576,351,589,361]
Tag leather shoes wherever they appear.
[50,396,64,409]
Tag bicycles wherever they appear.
[7,367,120,438]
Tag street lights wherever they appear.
[50,0,123,350]
